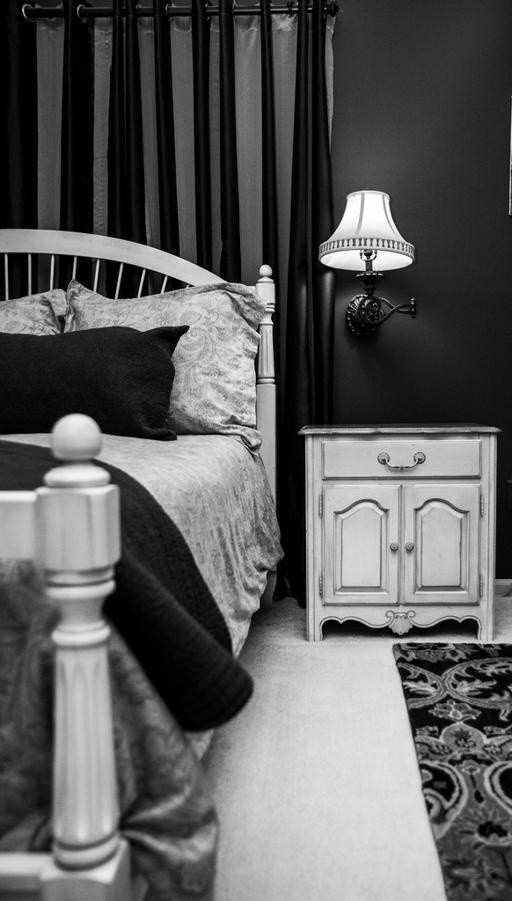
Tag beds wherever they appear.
[0,226,285,898]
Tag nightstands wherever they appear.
[300,422,498,642]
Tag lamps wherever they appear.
[317,190,417,345]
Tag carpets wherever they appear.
[393,643,512,900]
